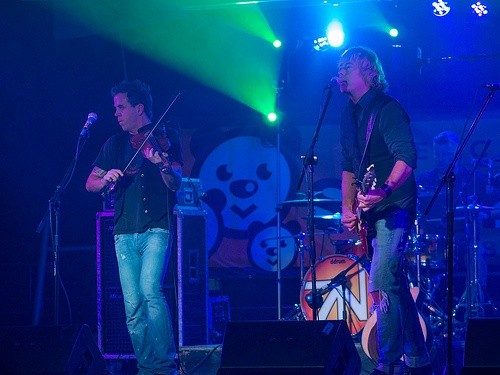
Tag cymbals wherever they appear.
[452,204,500,212]
[302,212,342,220]
[279,190,342,205]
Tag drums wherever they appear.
[299,255,374,338]
[406,235,447,270]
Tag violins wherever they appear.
[130,125,182,182]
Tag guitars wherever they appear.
[360,287,434,366]
[354,169,378,258]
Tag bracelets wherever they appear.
[377,182,392,198]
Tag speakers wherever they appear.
[462,318,500,375]
[0,323,108,375]
[215,320,363,375]
[96,208,210,361]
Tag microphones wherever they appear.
[328,76,341,88]
[260,241,267,248]
[81,112,98,135]
[480,82,500,90]
[306,292,323,309]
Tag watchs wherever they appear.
[160,161,173,175]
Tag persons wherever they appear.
[336,46,432,375]
[84,80,184,375]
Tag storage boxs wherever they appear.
[96,209,210,360]
[103,177,203,209]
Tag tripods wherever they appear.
[453,211,486,320]
